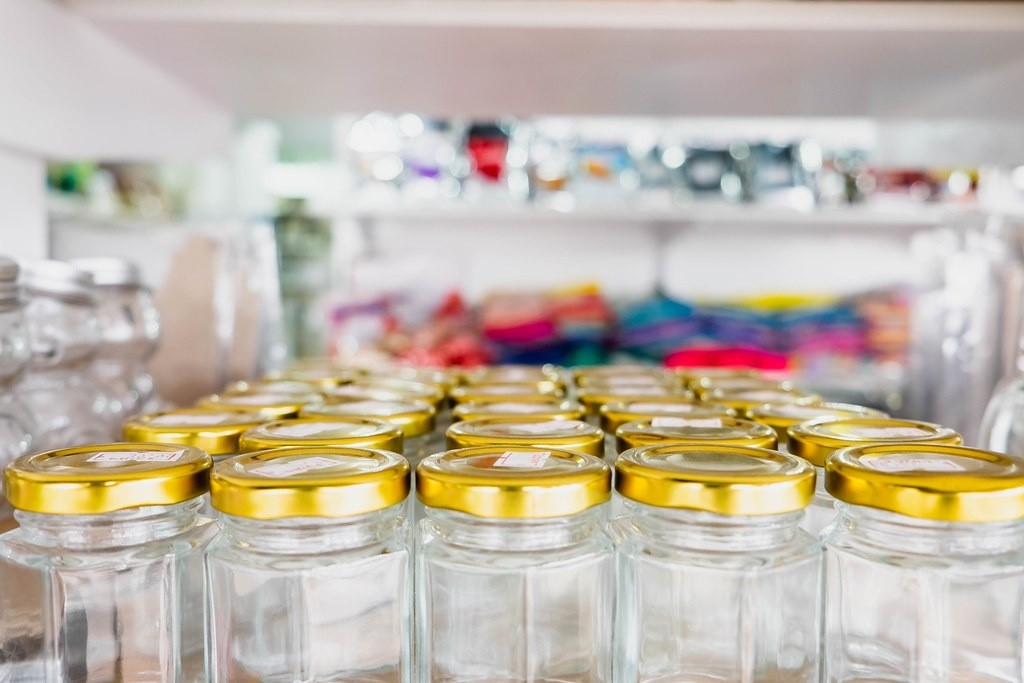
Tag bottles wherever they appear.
[409,446,619,683]
[119,360,970,654]
[606,443,824,681]
[2,251,172,453]
[824,438,1022,682]
[204,446,423,681]
[2,443,222,681]
